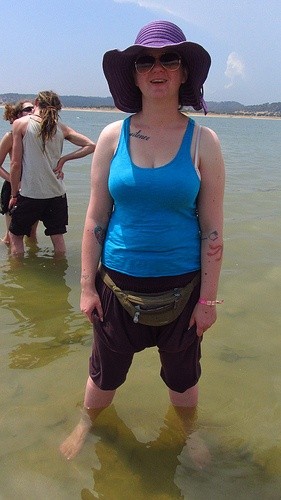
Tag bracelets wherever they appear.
[12,196,17,198]
[198,299,224,306]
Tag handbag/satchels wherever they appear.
[100,271,202,326]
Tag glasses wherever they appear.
[21,106,32,114]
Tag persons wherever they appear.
[59,21,225,471]
[8,90,96,254]
[0,100,39,244]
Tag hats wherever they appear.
[103,21,212,114]
